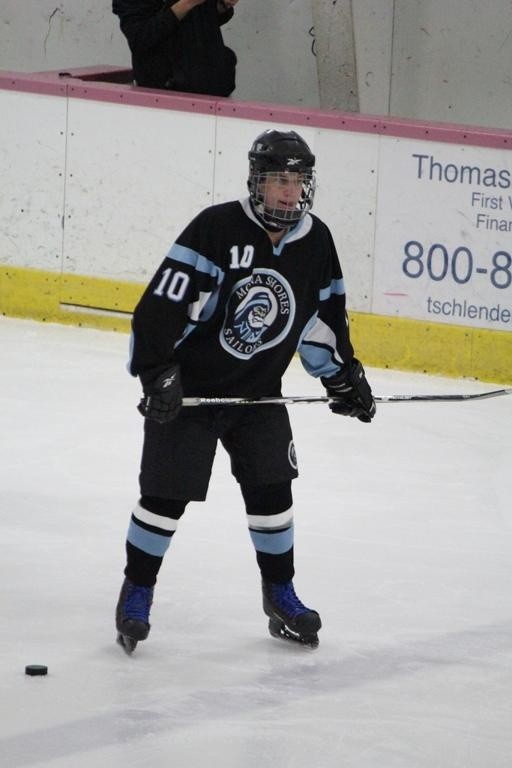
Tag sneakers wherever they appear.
[263,581,321,632]
[115,577,154,641]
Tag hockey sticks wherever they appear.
[180,387,512,408]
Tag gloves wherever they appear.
[321,358,376,423]
[137,366,183,424]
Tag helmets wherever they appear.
[248,128,318,232]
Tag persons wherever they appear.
[111,0,239,99]
[114,128,375,642]
